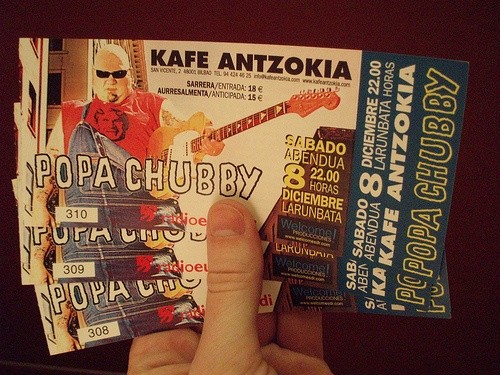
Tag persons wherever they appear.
[36,44,226,349]
[128,198,333,375]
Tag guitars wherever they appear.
[146,88,340,198]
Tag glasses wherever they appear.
[94,67,130,79]
